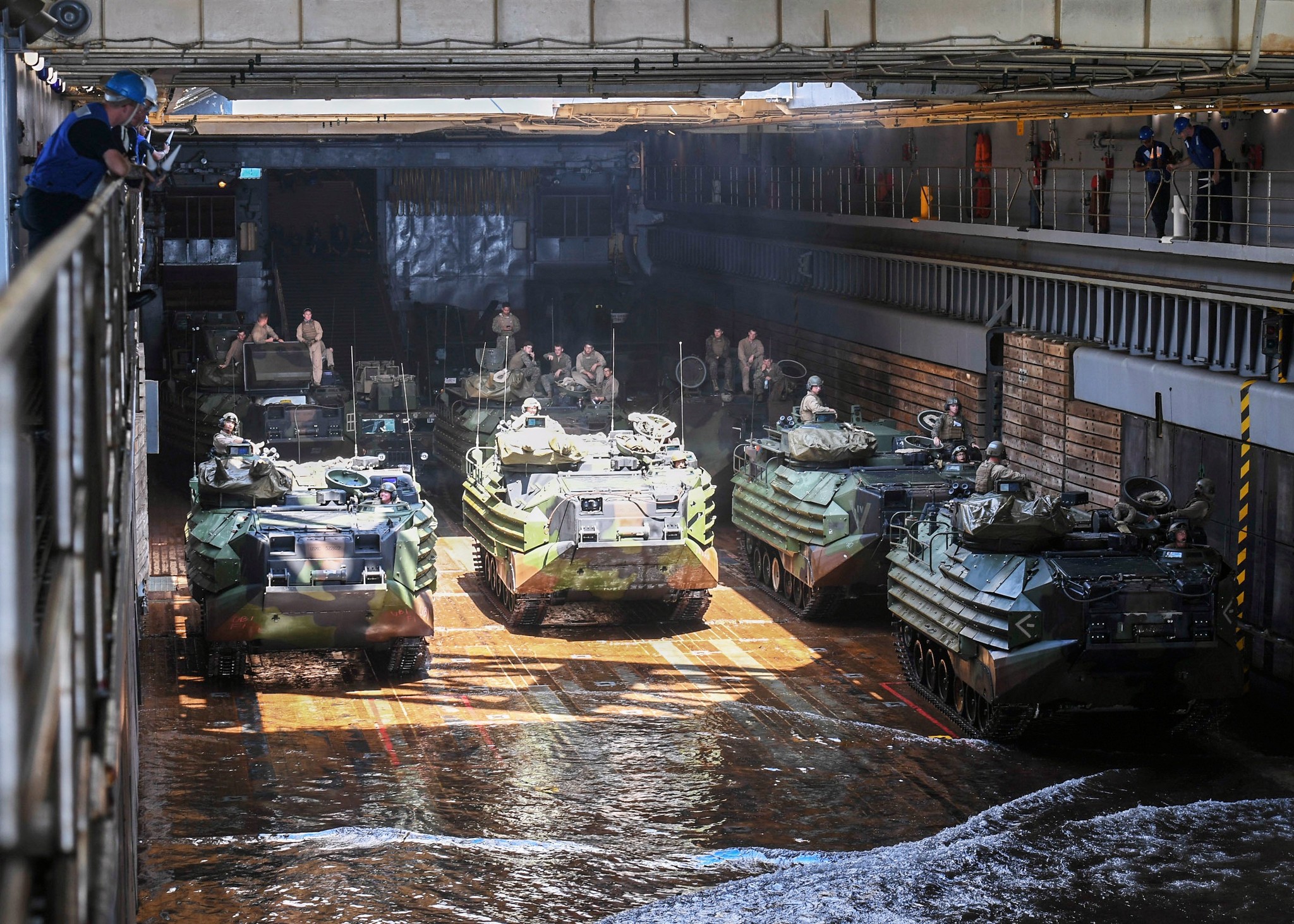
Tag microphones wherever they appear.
[522,410,534,416]
[1170,538,1186,543]
[954,458,965,461]
[218,424,230,431]
[1004,457,1010,467]
[808,389,821,393]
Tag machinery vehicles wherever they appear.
[156,310,349,476]
[461,414,722,631]
[338,356,436,460]
[186,452,438,684]
[727,401,982,617]
[428,346,631,515]
[878,477,1235,746]
[648,352,826,519]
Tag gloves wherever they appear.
[304,338,316,346]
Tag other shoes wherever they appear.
[313,382,321,387]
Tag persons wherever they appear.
[123,74,157,158]
[1146,475,1216,530]
[367,481,403,505]
[137,115,172,177]
[1132,126,1177,238]
[975,440,1028,496]
[1163,521,1193,548]
[19,69,162,310]
[1166,116,1232,241]
[191,289,974,473]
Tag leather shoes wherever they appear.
[127,289,157,313]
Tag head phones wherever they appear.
[521,397,542,415]
[945,397,962,411]
[951,446,967,463]
[806,375,824,393]
[218,412,239,432]
[984,441,1007,460]
[377,482,397,501]
[1167,522,1189,542]
[1194,480,1215,495]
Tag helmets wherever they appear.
[1196,478,1216,497]
[1168,522,1189,533]
[105,68,158,125]
[808,375,824,387]
[222,412,239,425]
[378,482,396,492]
[1138,125,1154,140]
[1173,117,1189,135]
[522,397,538,407]
[672,451,686,461]
[945,397,1005,457]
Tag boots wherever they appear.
[533,390,543,397]
[1154,219,1231,243]
[328,365,339,376]
[544,391,552,398]
[711,379,786,403]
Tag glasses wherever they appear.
[811,385,822,388]
[952,446,966,455]
[603,370,609,373]
[956,453,966,456]
[1140,139,1151,143]
[1174,522,1188,530]
[527,407,537,412]
[143,102,152,113]
[225,416,236,421]
[585,346,592,350]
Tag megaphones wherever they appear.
[48,0,93,37]
